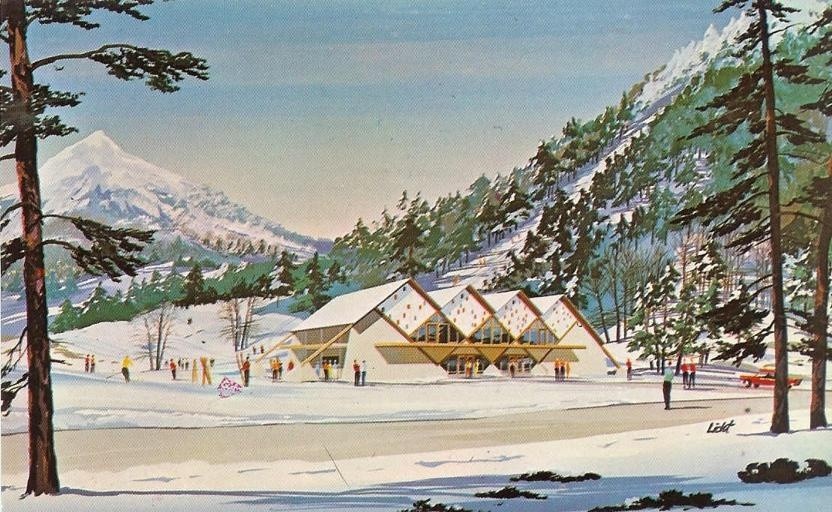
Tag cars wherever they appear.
[740,369,803,390]
